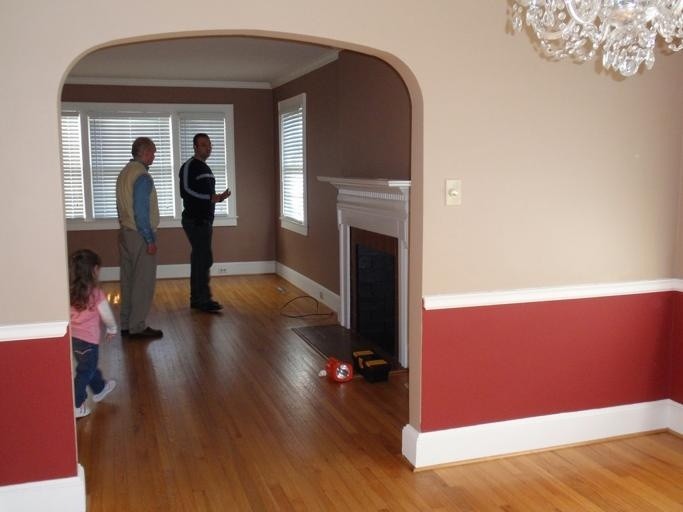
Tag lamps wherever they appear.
[504,0,683,78]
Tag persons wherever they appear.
[116,137,163,338]
[68,249,120,419]
[179,133,231,311]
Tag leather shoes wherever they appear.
[190,299,224,311]
[120,326,163,338]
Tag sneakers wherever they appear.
[74,379,116,418]
